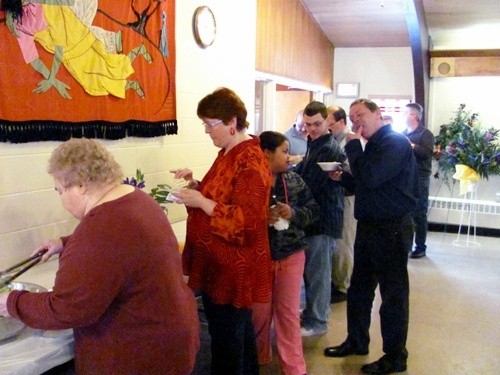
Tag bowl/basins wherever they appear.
[317,163,342,171]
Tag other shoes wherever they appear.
[300,326,328,336]
[331,290,347,303]
[411,250,425,258]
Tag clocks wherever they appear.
[194,5,216,48]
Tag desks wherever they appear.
[0,218,189,375]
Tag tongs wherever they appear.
[0,248,49,289]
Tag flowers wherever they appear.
[122,169,173,216]
[433,103,500,191]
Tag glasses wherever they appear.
[328,121,338,127]
[202,120,221,129]
[304,119,324,127]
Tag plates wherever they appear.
[0,282,48,341]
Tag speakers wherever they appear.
[429,57,455,78]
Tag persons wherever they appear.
[0,138,201,375]
[326,105,365,303]
[284,109,309,168]
[382,115,393,126]
[259,131,322,375]
[287,100,352,336]
[324,99,417,375]
[169,87,274,375]
[402,103,435,258]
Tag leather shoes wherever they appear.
[324,341,369,356]
[361,355,407,375]
